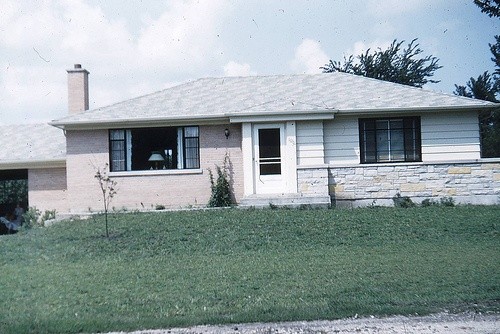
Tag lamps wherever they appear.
[224,128,230,139]
[148,154,167,170]
